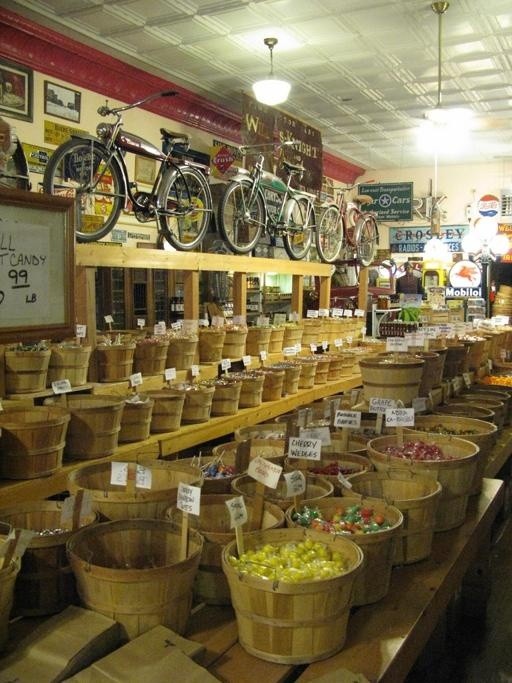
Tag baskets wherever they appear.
[379,319,417,337]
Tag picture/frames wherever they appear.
[43,80,82,123]
[0,57,34,124]
[162,141,210,196]
[134,153,157,184]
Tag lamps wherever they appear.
[421,2,455,122]
[252,37,291,107]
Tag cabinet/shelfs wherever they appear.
[0,239,511,682]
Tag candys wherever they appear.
[228,536,345,580]
[187,451,237,480]
[292,502,393,534]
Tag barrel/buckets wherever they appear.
[492,283,511,315]
[2,317,511,667]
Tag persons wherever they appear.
[395,262,425,294]
[332,258,351,287]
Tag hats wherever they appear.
[403,261,413,271]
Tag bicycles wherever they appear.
[0,117,31,192]
